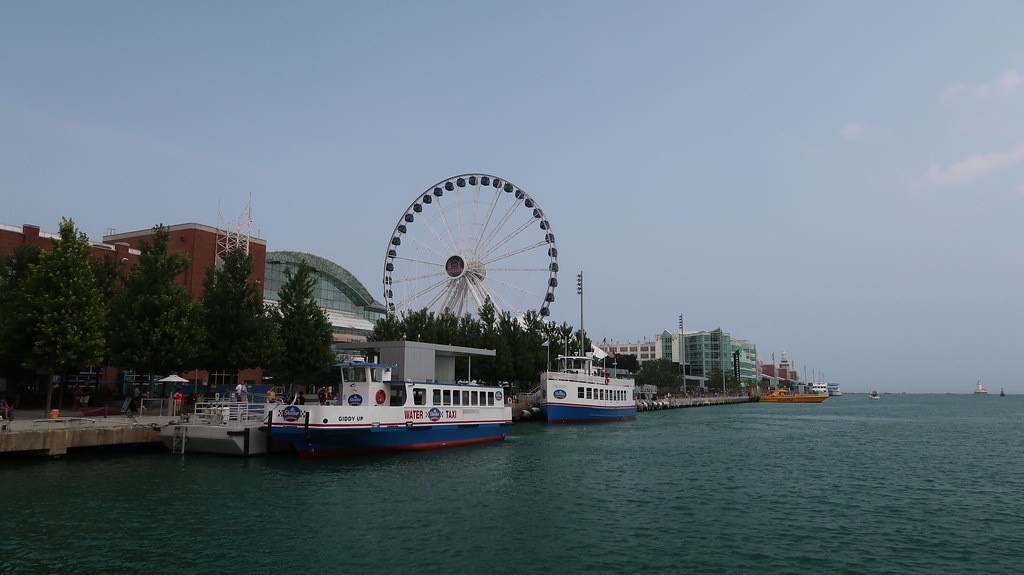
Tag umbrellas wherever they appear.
[158,375,190,383]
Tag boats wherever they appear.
[256,355,514,461]
[973,380,988,395]
[812,368,843,396]
[869,390,879,399]
[758,389,830,404]
[539,334,639,425]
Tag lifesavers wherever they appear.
[375,389,388,405]
[605,379,609,385]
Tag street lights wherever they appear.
[679,313,687,396]
[576,270,584,357]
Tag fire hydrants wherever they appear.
[50,409,59,423]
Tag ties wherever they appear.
[241,385,243,391]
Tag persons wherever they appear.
[667,391,751,398]
[71,382,330,417]
